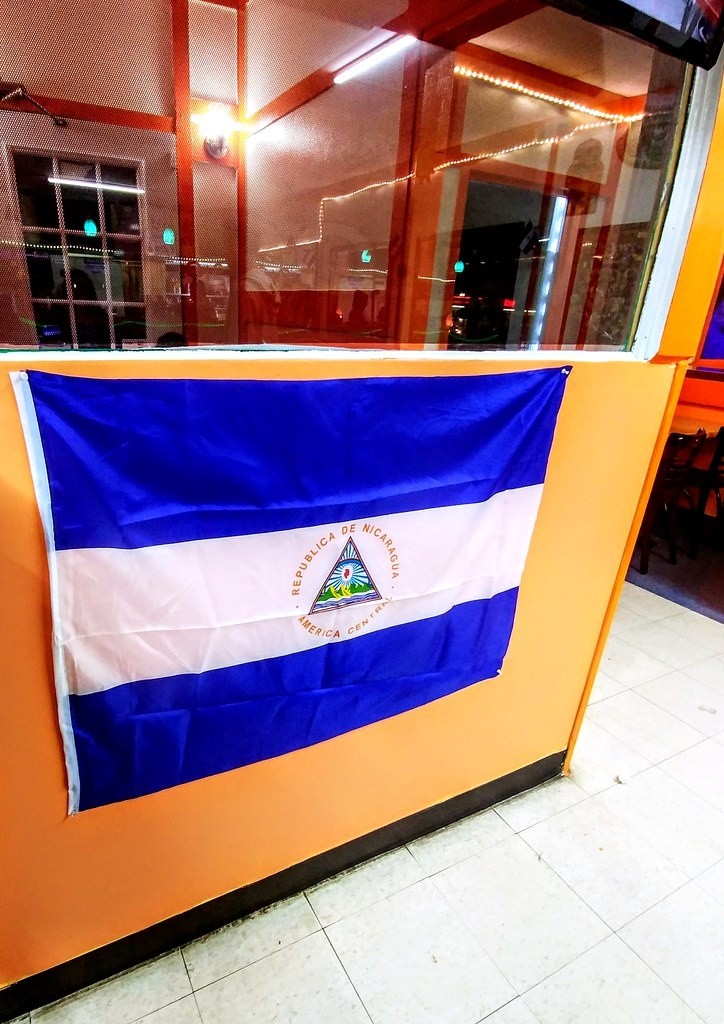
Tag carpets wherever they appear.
[626,504,724,621]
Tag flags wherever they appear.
[9,365,573,815]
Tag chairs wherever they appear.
[635,425,724,575]
